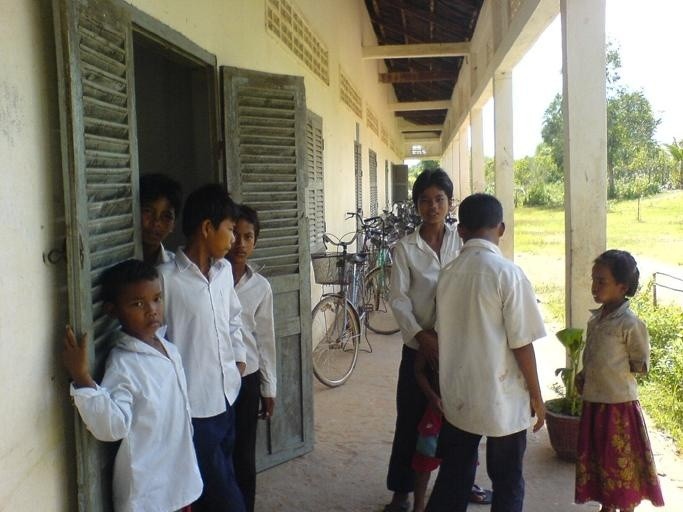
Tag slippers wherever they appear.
[468,483,494,506]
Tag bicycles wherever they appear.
[313,198,458,389]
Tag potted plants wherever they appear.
[542,326,587,462]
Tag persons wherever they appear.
[138,174,175,267]
[62,258,208,512]
[385,168,494,511]
[156,192,247,512]
[414,356,480,511]
[225,205,276,511]
[423,194,545,511]
[574,250,664,512]
